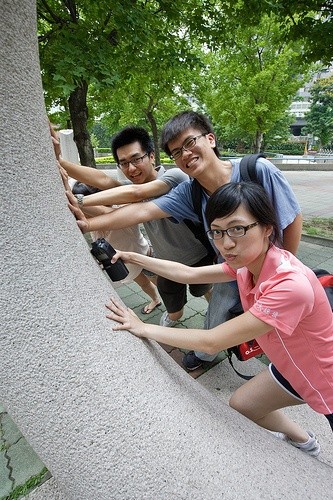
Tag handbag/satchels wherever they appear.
[228,269,333,361]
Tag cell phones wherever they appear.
[90,238,129,282]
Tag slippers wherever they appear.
[141,300,162,314]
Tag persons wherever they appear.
[65,110,303,371]
[96,182,333,458]
[49,122,213,328]
[72,178,162,315]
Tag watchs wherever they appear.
[74,194,84,206]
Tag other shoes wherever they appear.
[272,431,321,457]
[160,311,179,327]
[182,350,204,371]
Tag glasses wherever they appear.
[116,154,148,170]
[170,135,203,161]
[206,222,259,240]
